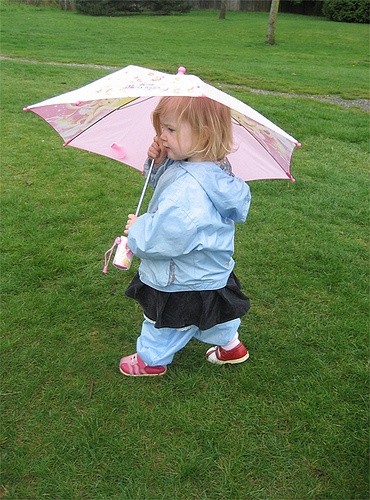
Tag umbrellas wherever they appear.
[22,62,303,275]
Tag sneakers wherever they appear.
[204,341,250,365]
[119,352,168,376]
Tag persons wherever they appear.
[118,96,253,377]
